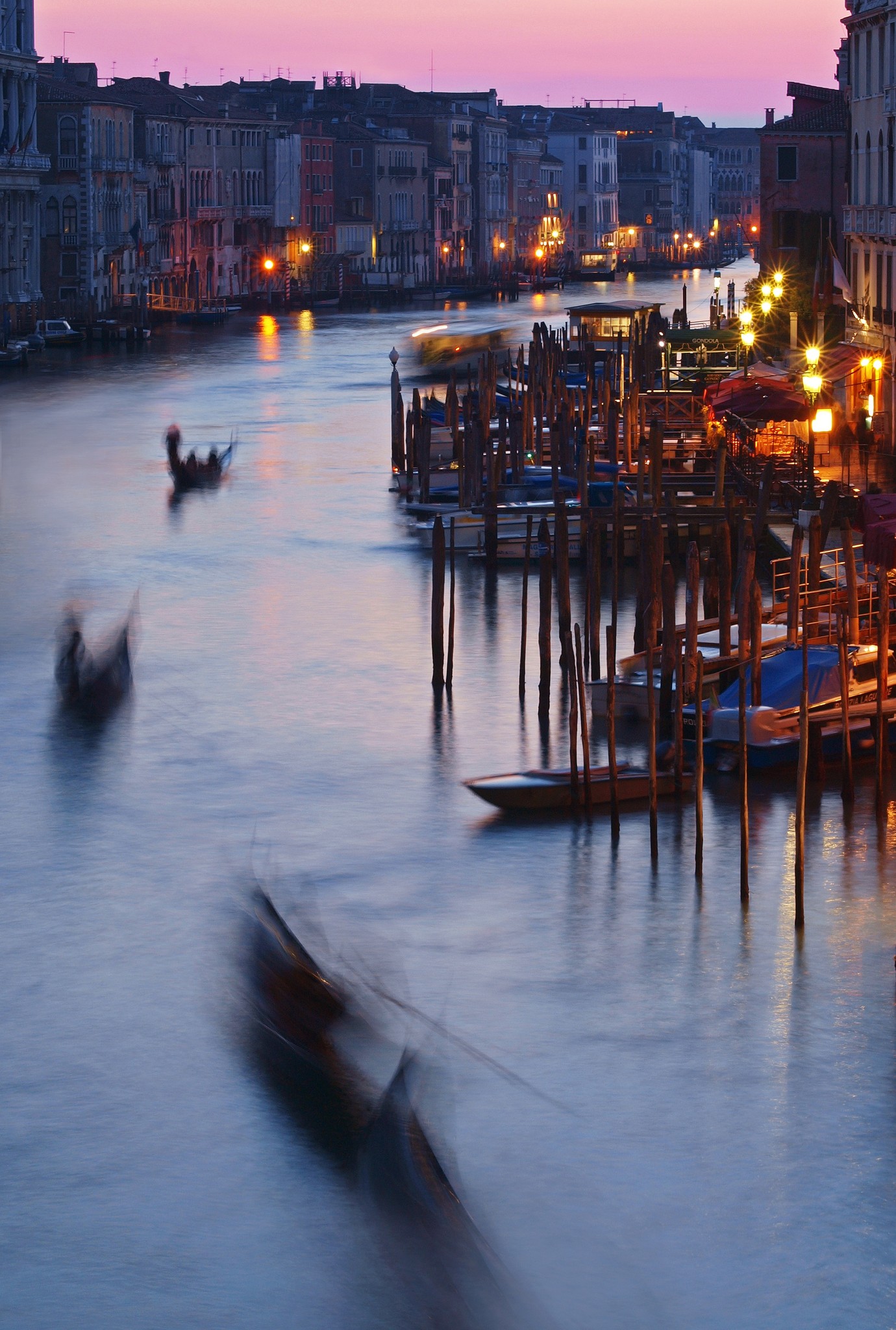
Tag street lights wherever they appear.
[798,347,821,529]
[762,272,783,363]
[265,260,274,314]
[713,271,721,306]
[740,311,754,377]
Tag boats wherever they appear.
[249,886,474,1221]
[427,348,511,377]
[0,320,150,362]
[404,288,476,300]
[484,271,562,290]
[55,627,132,718]
[393,348,702,558]
[168,442,232,488]
[669,643,896,771]
[462,762,696,810]
[279,297,340,309]
[177,307,242,324]
